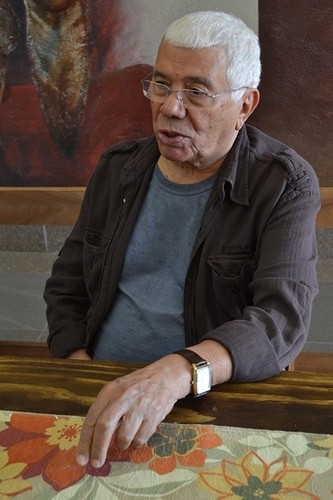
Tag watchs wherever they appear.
[171,347,213,399]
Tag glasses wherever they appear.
[141,73,249,110]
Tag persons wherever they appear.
[39,9,323,469]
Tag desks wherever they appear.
[0,355,333,500]
